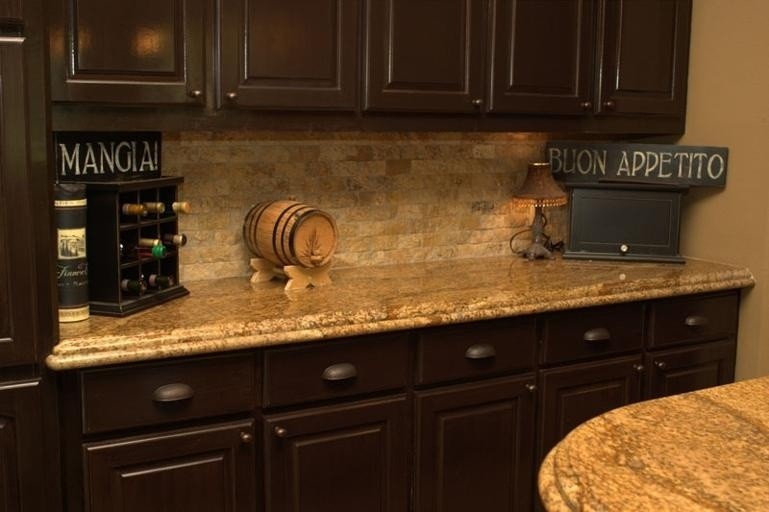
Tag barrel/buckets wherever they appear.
[243,200,337,267]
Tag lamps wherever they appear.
[512,161,568,264]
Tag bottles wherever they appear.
[118,200,192,295]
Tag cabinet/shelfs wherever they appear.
[45,0,362,131]
[538,337,737,468]
[79,392,413,512]
[361,0,491,133]
[80,289,742,439]
[413,369,539,512]
[57,175,189,315]
[490,1,691,134]
